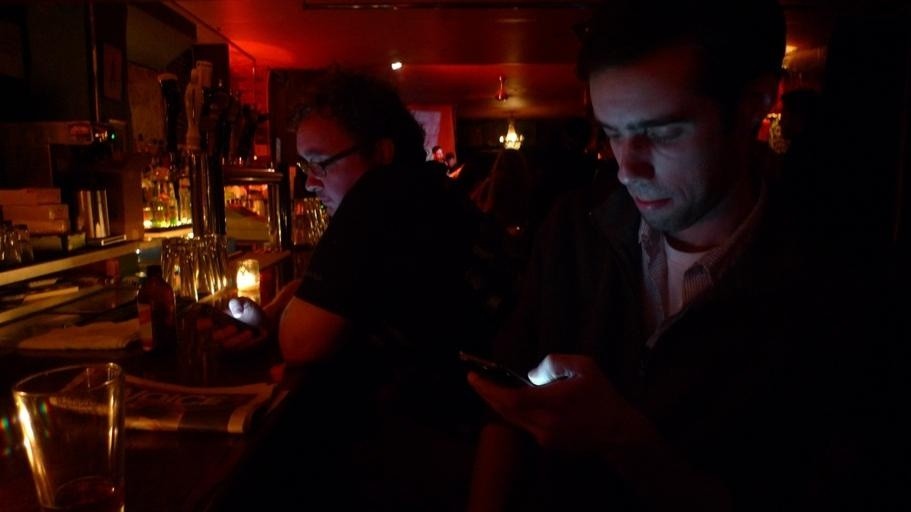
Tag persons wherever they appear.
[467,0,911,512]
[574,161,639,357]
[212,69,491,511]
[780,88,818,140]
[432,113,601,343]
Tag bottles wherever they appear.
[291,198,329,247]
[143,182,180,227]
[137,264,176,350]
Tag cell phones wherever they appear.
[459,352,536,388]
[199,301,263,336]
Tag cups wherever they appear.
[11,361,124,512]
[159,236,232,303]
[0,225,33,266]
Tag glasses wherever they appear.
[295,145,360,178]
